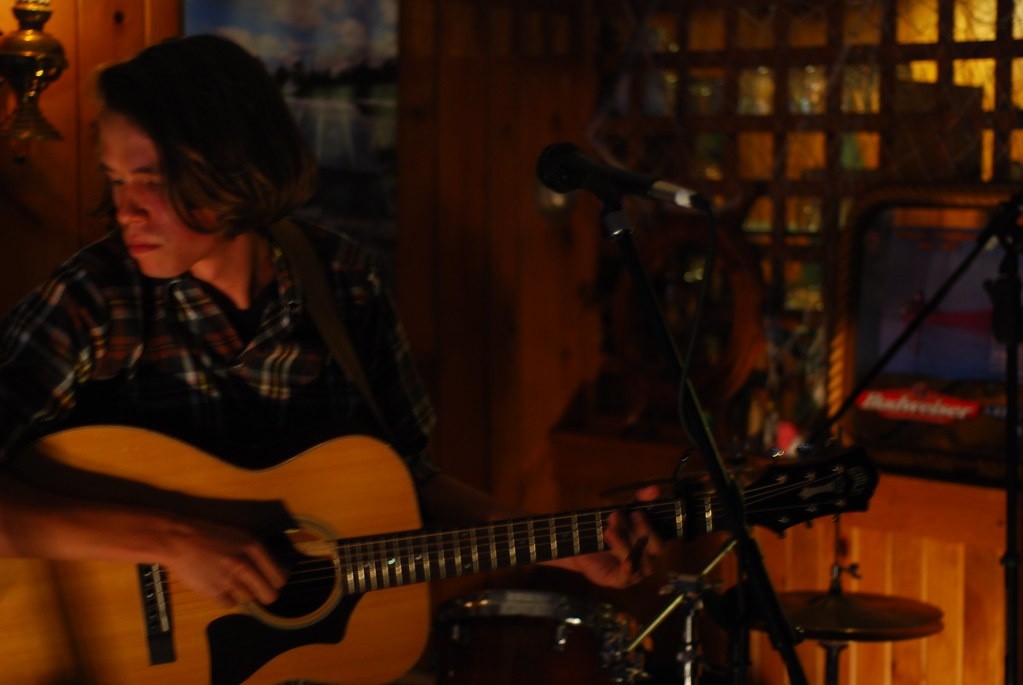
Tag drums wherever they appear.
[421,588,655,685]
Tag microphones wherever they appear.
[534,141,710,210]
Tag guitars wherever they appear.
[1,422,882,685]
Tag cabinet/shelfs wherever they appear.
[553,440,1008,685]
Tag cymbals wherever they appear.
[735,585,947,645]
[592,467,739,497]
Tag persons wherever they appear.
[0,34,675,685]
